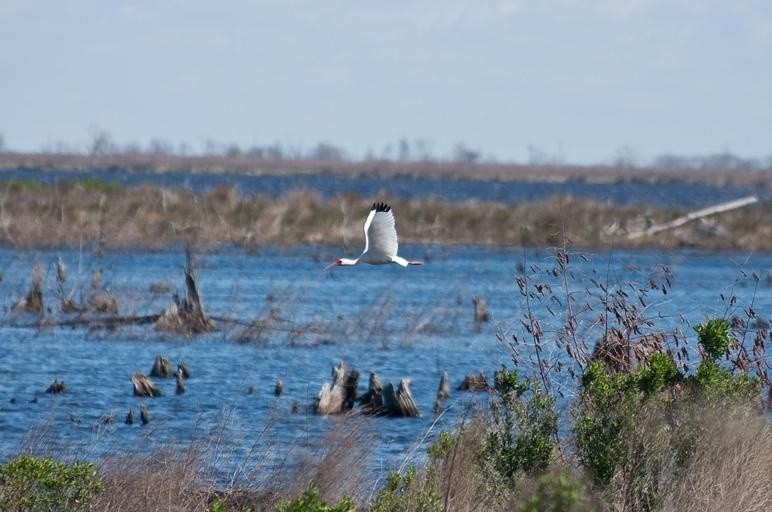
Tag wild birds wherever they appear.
[320,201,425,273]
